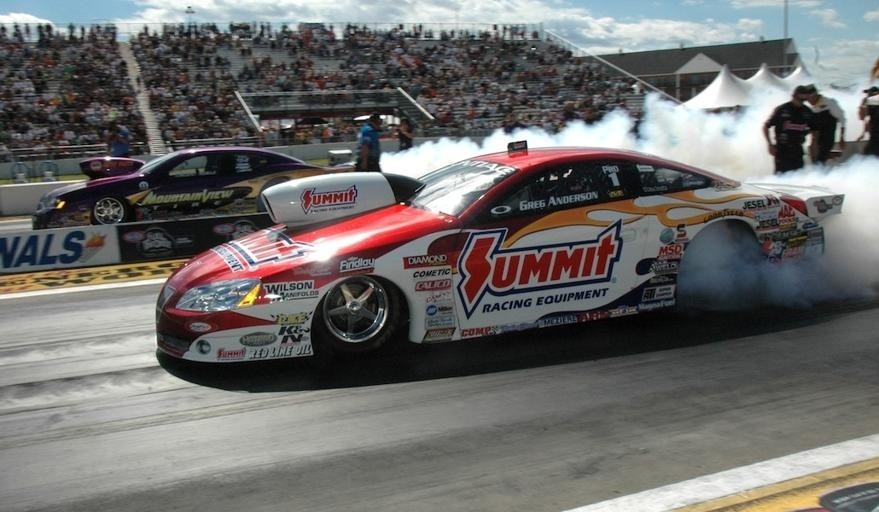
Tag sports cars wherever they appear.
[23,145,356,232]
[152,141,850,386]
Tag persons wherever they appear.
[762,85,815,174]
[806,84,846,164]
[860,86,879,156]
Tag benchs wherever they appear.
[1,36,646,164]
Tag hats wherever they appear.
[863,86,878,93]
[805,84,823,96]
[794,85,809,98]
[366,113,383,126]
